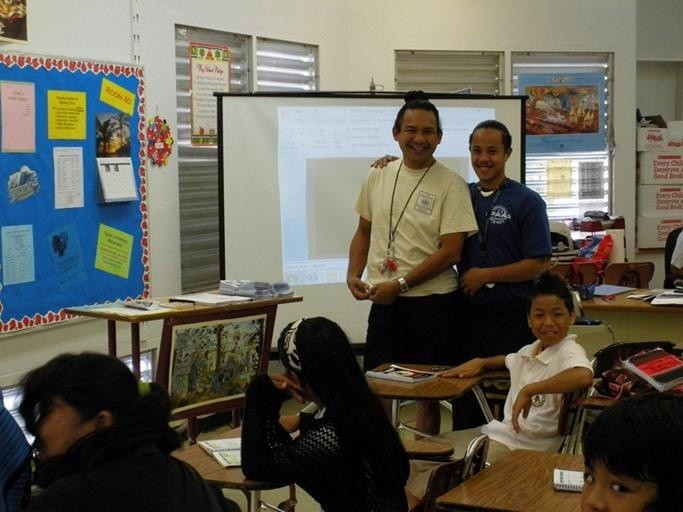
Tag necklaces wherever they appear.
[377,158,437,278]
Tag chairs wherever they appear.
[663,224,683,287]
[402,432,487,511]
[605,262,655,289]
[483,361,592,455]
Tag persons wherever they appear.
[17,353,241,511]
[369,120,555,430]
[405,277,595,511]
[580,392,682,511]
[104,119,129,153]
[0,390,32,511]
[345,91,480,369]
[240,316,411,511]
[670,231,683,278]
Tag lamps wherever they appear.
[579,219,604,239]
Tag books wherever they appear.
[366,363,438,383]
[592,283,637,296]
[553,467,585,493]
[622,347,683,394]
[217,276,296,302]
[196,437,241,468]
[626,284,683,306]
[169,291,254,307]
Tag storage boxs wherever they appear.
[635,115,682,251]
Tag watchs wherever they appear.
[398,277,408,293]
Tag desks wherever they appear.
[573,387,617,456]
[578,283,682,361]
[63,289,303,445]
[362,361,510,438]
[165,414,455,511]
[437,447,584,510]
